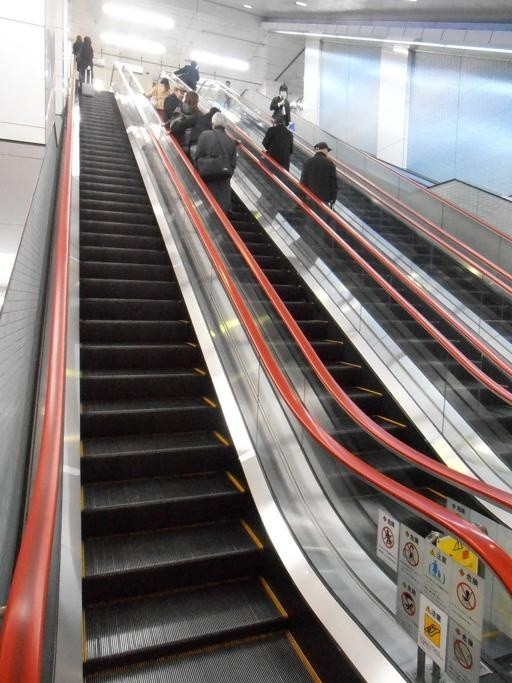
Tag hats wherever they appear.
[314,142,331,152]
[174,86,187,92]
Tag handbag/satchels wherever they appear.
[197,156,233,181]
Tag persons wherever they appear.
[163,85,186,123]
[173,59,199,92]
[269,83,291,128]
[187,105,222,164]
[194,111,238,214]
[298,139,338,207]
[223,80,232,107]
[72,34,84,56]
[168,90,214,157]
[143,77,173,116]
[262,115,293,171]
[76,35,94,85]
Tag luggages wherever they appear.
[81,82,95,97]
